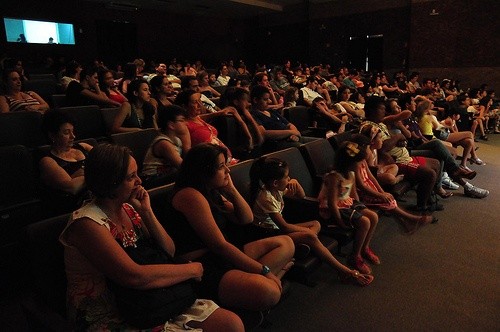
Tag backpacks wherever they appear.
[105,238,195,330]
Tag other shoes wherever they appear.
[442,177,459,189]
[403,215,439,236]
[355,257,372,274]
[494,129,500,134]
[470,145,479,154]
[470,157,483,166]
[344,269,374,287]
[459,164,476,180]
[480,134,488,140]
[364,247,381,265]
[484,129,492,134]
[464,185,490,198]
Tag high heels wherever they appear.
[434,189,454,199]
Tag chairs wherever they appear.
[0,69,412,332]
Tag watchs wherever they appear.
[261,265,270,276]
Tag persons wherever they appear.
[1,51,500,332]
